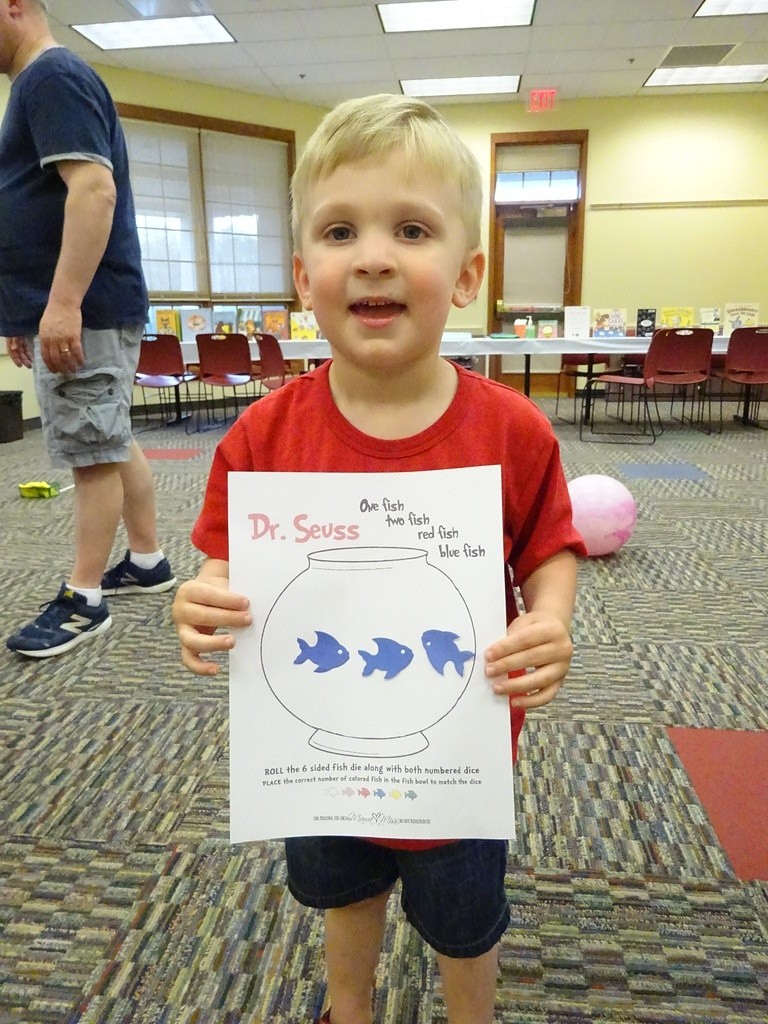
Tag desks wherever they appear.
[167,336,760,428]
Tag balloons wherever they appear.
[565,473,638,556]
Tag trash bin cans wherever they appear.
[0,390,23,443]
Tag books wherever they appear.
[236,308,262,339]
[700,307,721,334]
[536,319,558,339]
[661,308,696,328]
[180,308,212,341]
[290,312,327,337]
[155,310,181,342]
[592,309,627,339]
[723,303,761,337]
[261,310,290,341]
[637,309,657,336]
[213,313,237,336]
[564,305,590,338]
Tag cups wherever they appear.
[514,325,526,338]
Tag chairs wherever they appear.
[128,332,331,435]
[554,326,768,446]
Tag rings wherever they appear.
[62,349,70,353]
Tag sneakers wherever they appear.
[5,582,113,659]
[96,549,177,596]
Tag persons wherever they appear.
[0,0,176,660]
[173,93,586,1024]
